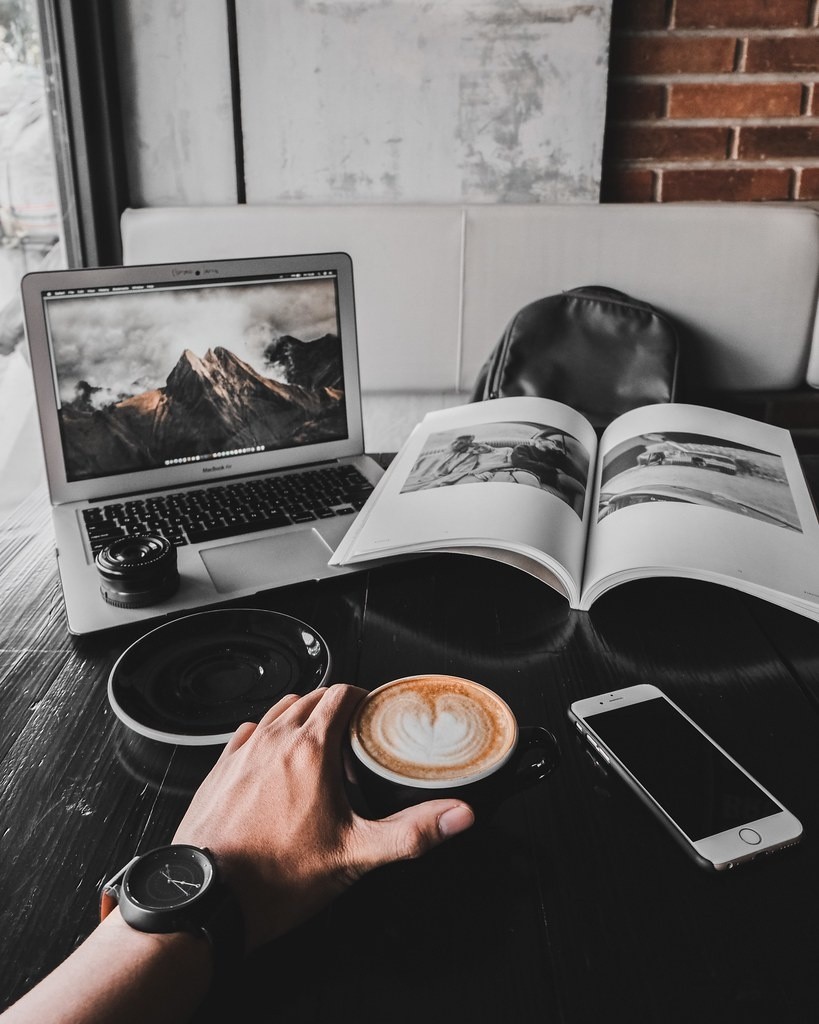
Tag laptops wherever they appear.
[20,251,433,637]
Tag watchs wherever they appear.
[98,843,250,1002]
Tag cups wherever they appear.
[342,673,563,836]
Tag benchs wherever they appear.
[403,440,572,506]
[120,198,819,514]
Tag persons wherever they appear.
[510,436,586,514]
[0,681,476,1024]
[453,438,517,487]
[400,430,478,495]
[613,448,708,505]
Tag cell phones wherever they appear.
[568,684,804,874]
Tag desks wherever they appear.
[0,455,819,1024]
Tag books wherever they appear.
[326,396,819,624]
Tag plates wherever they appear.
[105,608,333,747]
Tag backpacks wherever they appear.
[468,286,692,442]
